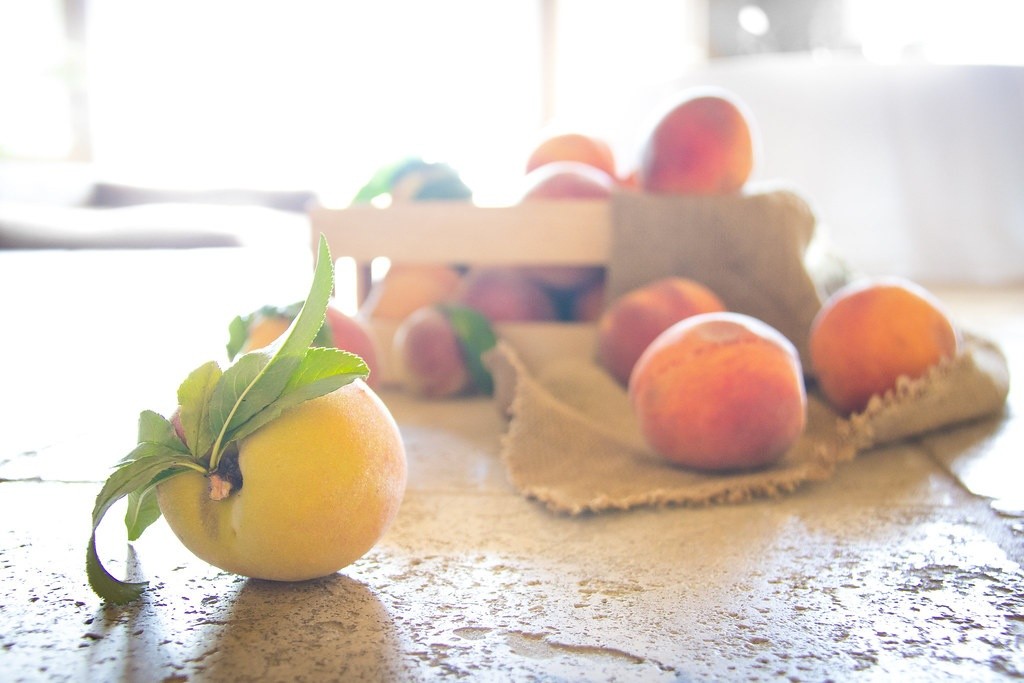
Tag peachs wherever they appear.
[150,96,959,581]
